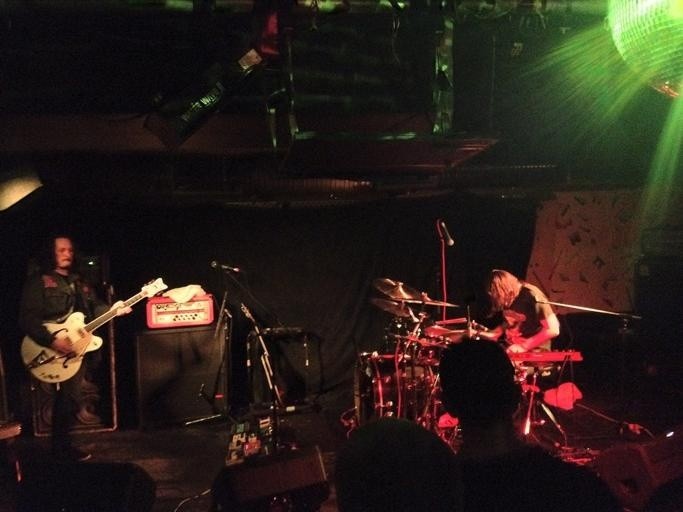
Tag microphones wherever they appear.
[211,261,240,272]
[466,304,473,337]
[440,220,455,246]
[198,383,206,398]
[527,295,538,304]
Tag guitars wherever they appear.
[20,279,169,385]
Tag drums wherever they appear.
[403,336,447,366]
[353,352,442,434]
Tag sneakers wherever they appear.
[67,446,92,462]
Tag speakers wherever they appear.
[25,252,120,437]
[210,448,331,512]
[583,435,683,512]
[12,462,156,512]
[129,324,231,430]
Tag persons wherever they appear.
[466,272,561,377]
[19,234,134,463]
[334,416,466,512]
[441,340,625,511]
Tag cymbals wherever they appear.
[372,277,422,302]
[372,299,413,318]
[443,328,497,343]
[405,298,461,308]
